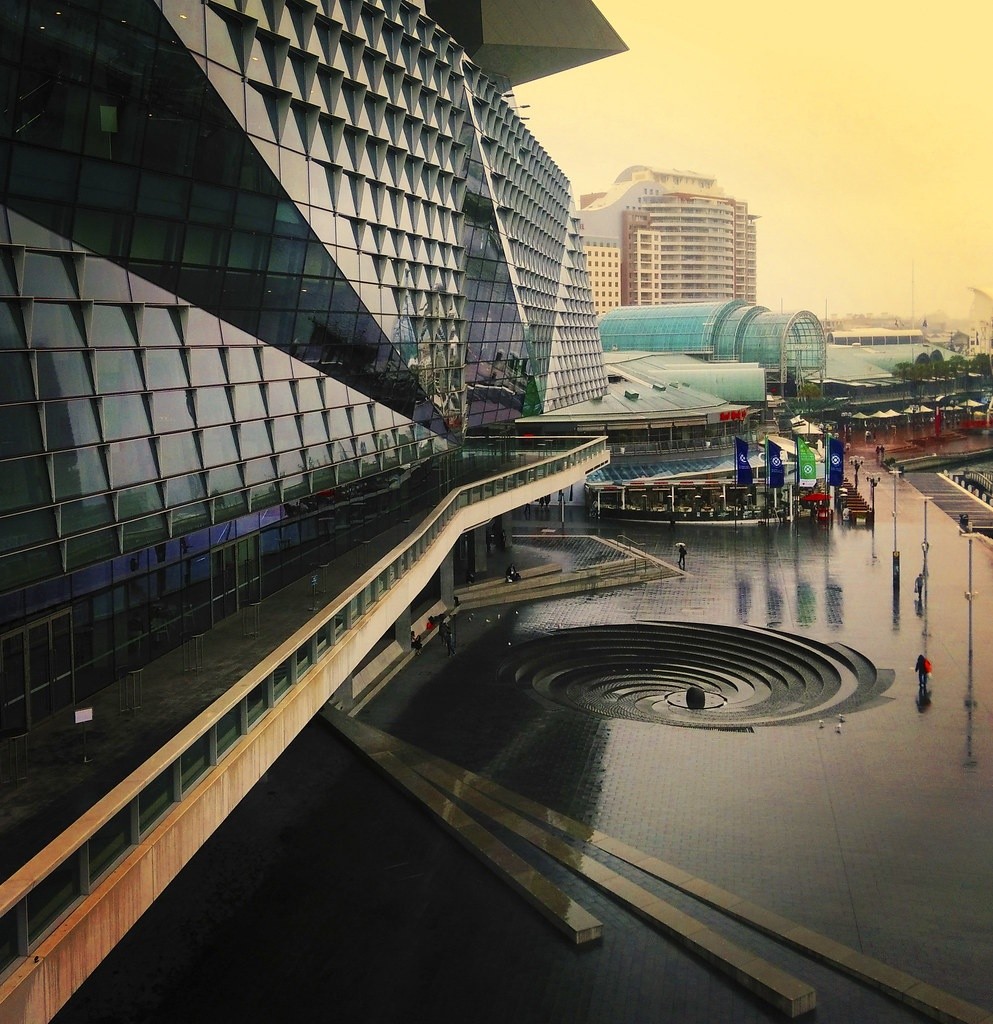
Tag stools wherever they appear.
[537,444,546,461]
[351,503,367,529]
[488,443,496,461]
[310,561,330,599]
[544,439,553,457]
[117,665,144,718]
[353,538,372,571]
[240,599,262,639]
[0,727,31,788]
[319,517,335,546]
[154,604,165,622]
[397,518,410,540]
[178,603,196,632]
[182,629,207,677]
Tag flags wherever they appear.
[767,439,785,489]
[736,438,753,487]
[827,434,844,487]
[797,436,816,479]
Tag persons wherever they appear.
[438,619,457,657]
[915,654,930,688]
[843,421,855,451]
[677,545,688,565]
[863,416,898,442]
[819,421,840,439]
[907,412,961,431]
[914,573,925,600]
[411,631,422,657]
[505,563,518,582]
[874,445,885,458]
[669,519,676,532]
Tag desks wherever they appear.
[838,478,873,525]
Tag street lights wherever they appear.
[848,454,869,490]
[865,472,880,517]
[915,495,936,636]
[957,513,980,705]
[889,468,903,612]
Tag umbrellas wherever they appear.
[801,493,833,520]
[901,404,933,423]
[940,398,985,422]
[676,543,686,547]
[851,409,906,426]
